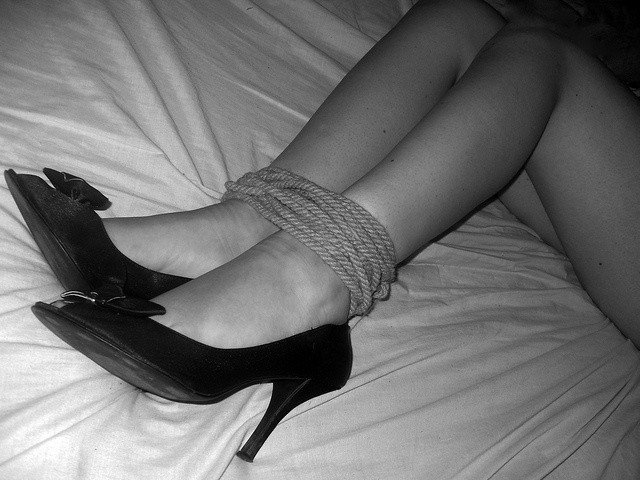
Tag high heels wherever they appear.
[32,290,353,462]
[5,167,194,300]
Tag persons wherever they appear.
[4,0,640,464]
